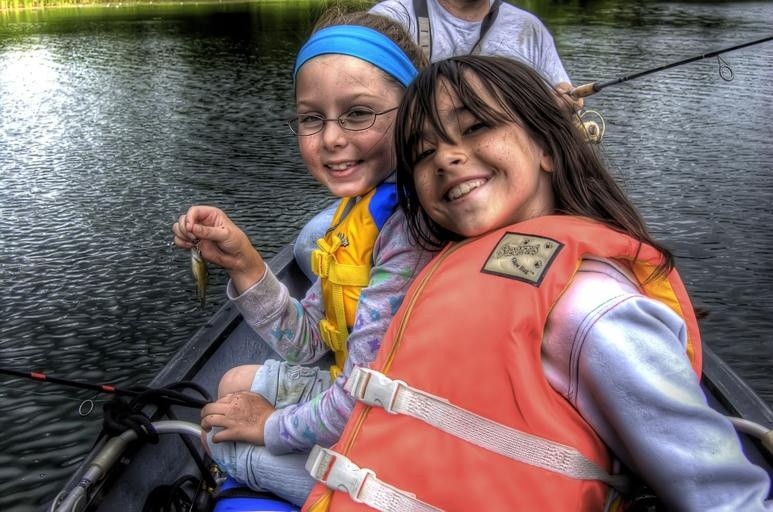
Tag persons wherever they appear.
[172,2,450,508]
[298,53,772,512]
[288,1,590,285]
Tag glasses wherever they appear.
[284,103,400,137]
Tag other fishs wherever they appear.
[190,246,210,310]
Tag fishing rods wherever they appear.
[570,37,773,144]
[0,368,202,409]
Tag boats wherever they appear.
[49,197,769,510]
